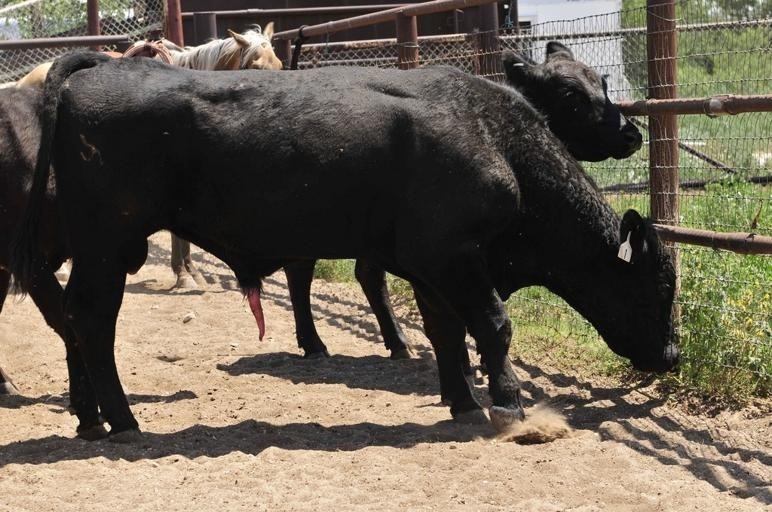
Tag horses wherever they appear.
[12,20,284,290]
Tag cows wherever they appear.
[1,41,681,446]
